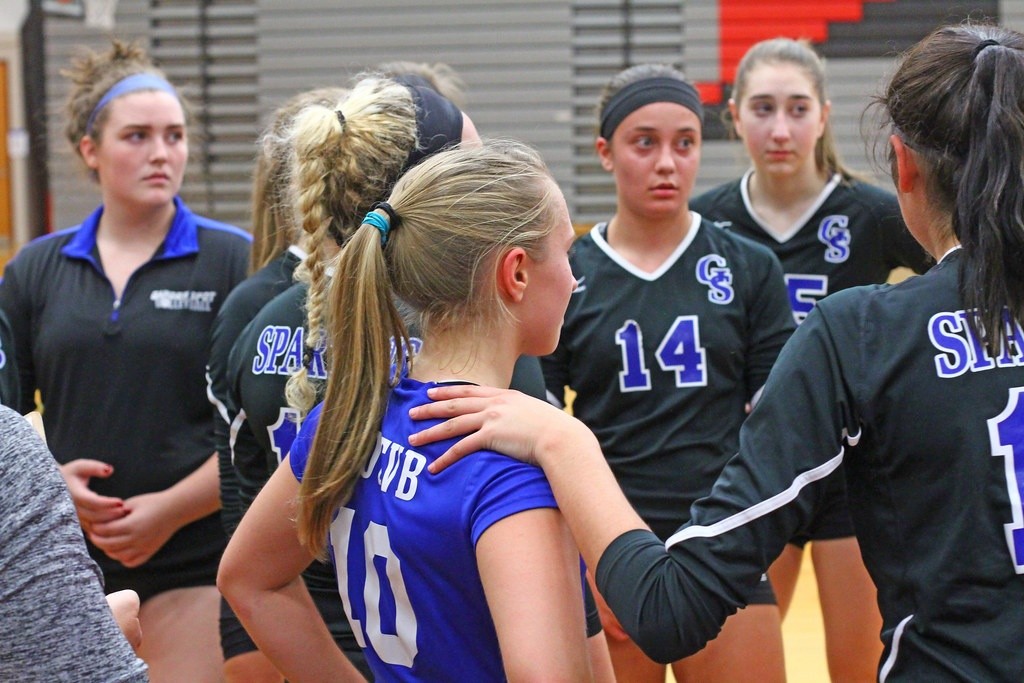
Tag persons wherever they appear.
[685,38,937,683]
[216,138,593,683]
[407,21,1024,683]
[0,40,491,683]
[537,63,805,683]
